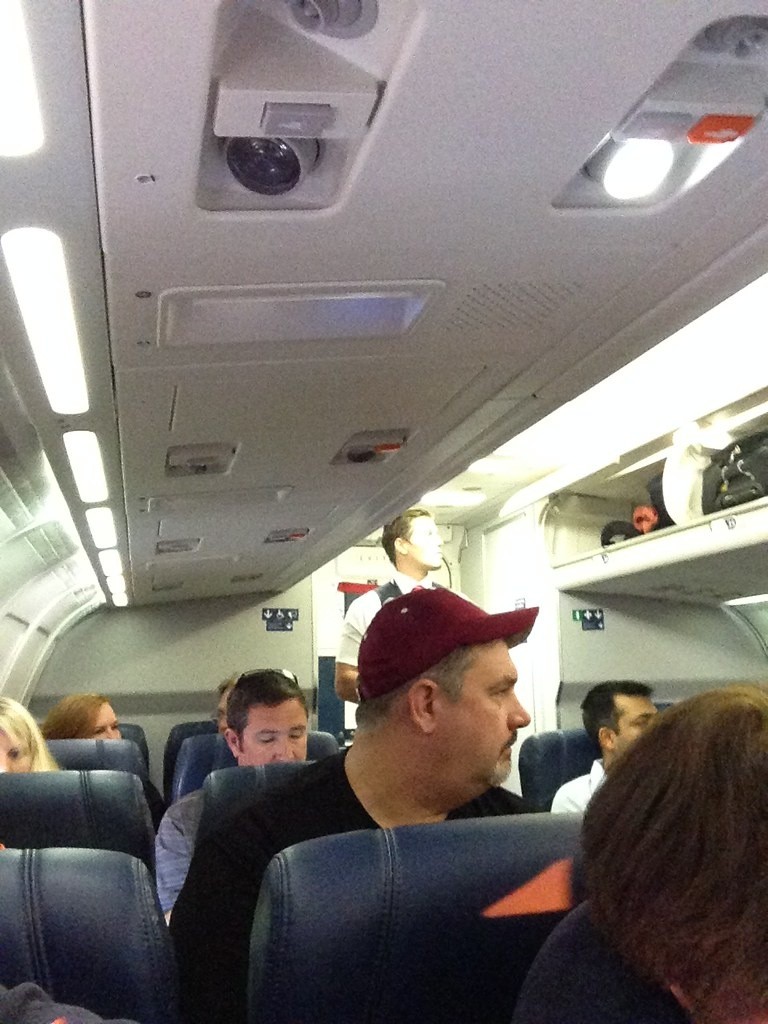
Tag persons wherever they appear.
[333,504,479,707]
[154,665,318,932]
[211,669,243,738]
[0,980,148,1024]
[509,687,768,1023]
[39,692,168,840]
[550,679,660,815]
[166,584,550,1024]
[0,693,64,776]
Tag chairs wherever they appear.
[0,715,598,1024]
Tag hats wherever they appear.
[355,593,537,704]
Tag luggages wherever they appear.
[705,430,766,514]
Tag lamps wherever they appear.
[585,138,677,201]
[222,131,322,200]
